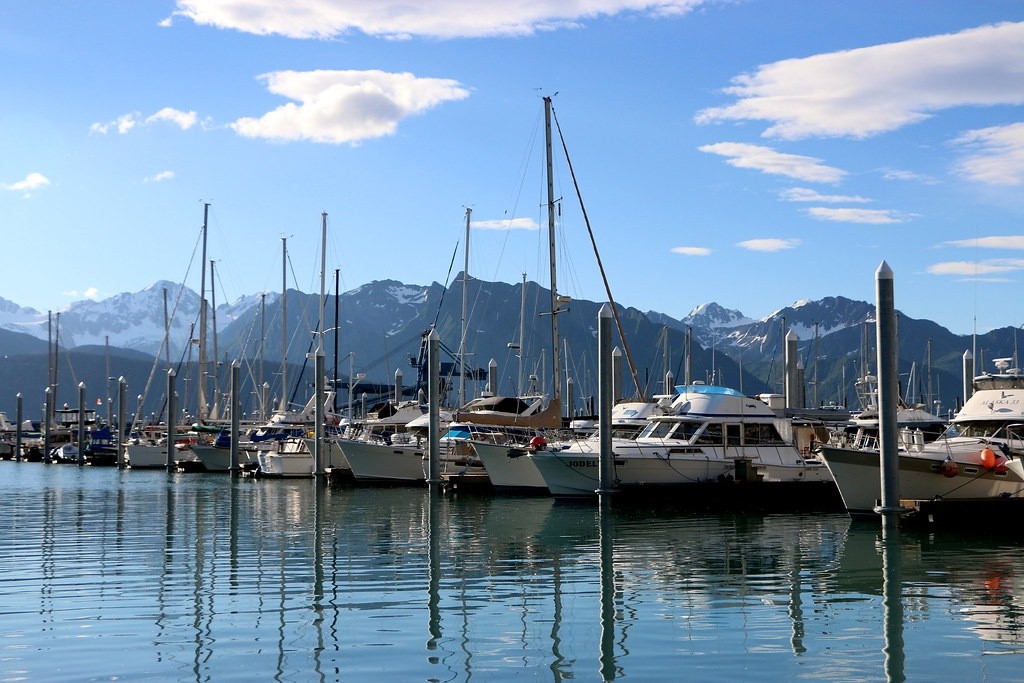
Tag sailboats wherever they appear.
[1,88,1024,499]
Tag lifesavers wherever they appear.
[530,436,546,448]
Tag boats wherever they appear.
[808,312,1024,513]
